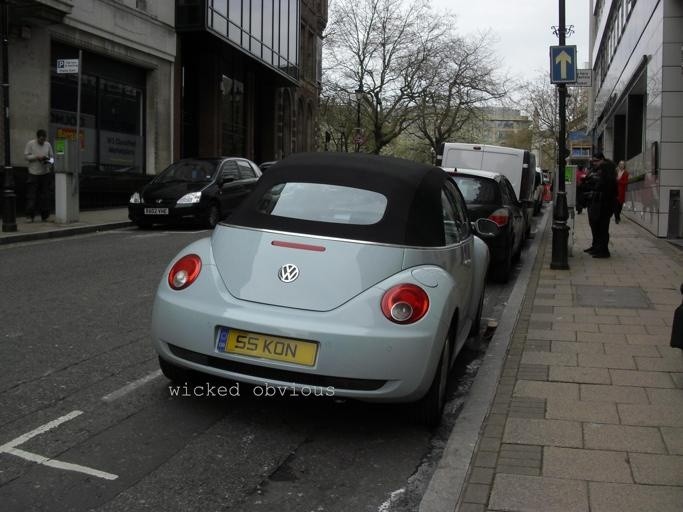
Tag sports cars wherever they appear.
[148,148,501,432]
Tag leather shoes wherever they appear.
[584,246,610,258]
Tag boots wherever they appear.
[615,212,621,224]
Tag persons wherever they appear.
[576,150,630,259]
[24,129,52,223]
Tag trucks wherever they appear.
[441,141,537,239]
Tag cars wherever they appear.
[126,155,265,230]
[441,165,536,284]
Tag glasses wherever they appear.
[590,159,600,163]
